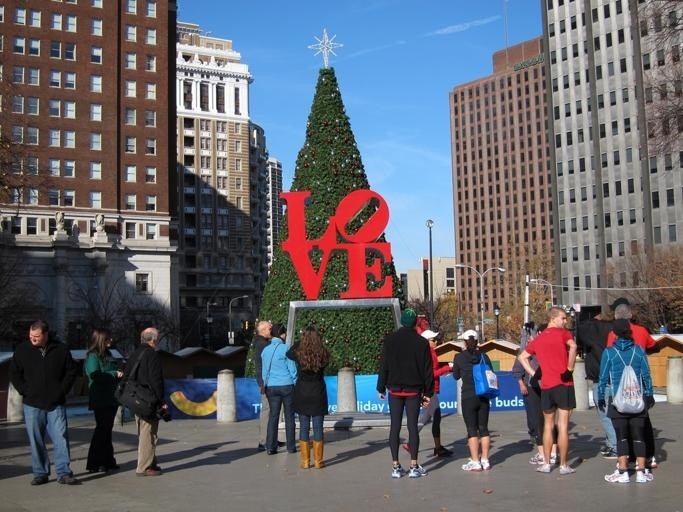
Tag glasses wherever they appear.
[429,339,436,342]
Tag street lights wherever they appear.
[426,218,434,317]
[494,305,500,338]
[454,263,507,336]
[205,301,219,347]
[228,295,249,344]
[527,278,553,310]
[475,325,480,344]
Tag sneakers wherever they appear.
[408,464,428,478]
[549,454,558,464]
[635,467,654,483]
[600,448,610,454]
[604,469,631,483]
[558,464,577,475]
[401,440,412,455]
[391,462,408,479]
[461,460,482,472]
[481,459,491,470]
[529,453,544,465]
[647,455,658,470]
[601,449,618,460]
[535,463,552,474]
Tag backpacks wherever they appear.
[610,343,644,413]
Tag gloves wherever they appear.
[645,395,655,410]
[560,367,574,382]
[155,405,173,423]
[531,365,542,381]
[598,399,607,412]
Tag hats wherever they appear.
[270,323,287,337]
[609,296,631,312]
[462,329,478,341]
[420,329,439,341]
[611,317,633,338]
[399,306,419,328]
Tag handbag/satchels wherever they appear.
[472,352,501,400]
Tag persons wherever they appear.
[512,322,558,465]
[112,327,171,476]
[376,308,434,478]
[286,326,330,469]
[578,309,631,460]
[84,329,123,473]
[607,298,658,468]
[9,320,81,485]
[260,324,298,455]
[254,321,285,452]
[453,330,493,471]
[597,320,654,483]
[518,307,577,475]
[402,330,453,459]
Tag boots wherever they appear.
[298,439,311,470]
[312,439,326,469]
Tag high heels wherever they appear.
[433,446,453,458]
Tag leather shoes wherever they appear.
[135,464,163,476]
[257,444,267,451]
[266,448,279,455]
[287,448,298,454]
[30,475,50,486]
[278,441,286,448]
[57,472,82,485]
[88,463,120,474]
